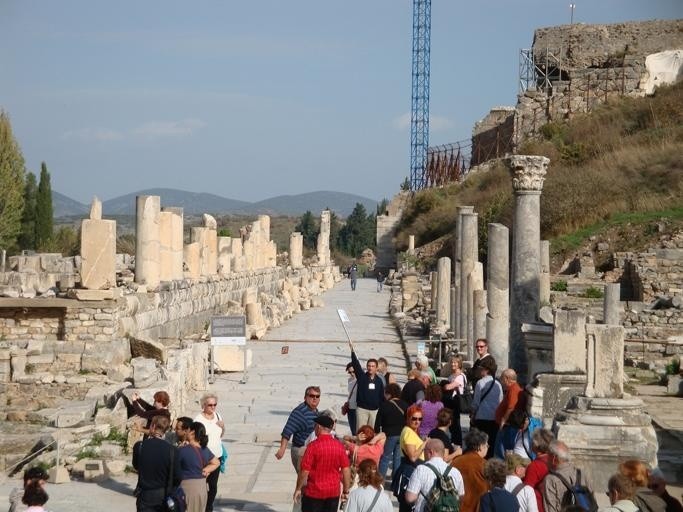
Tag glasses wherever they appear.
[207,403,217,407]
[411,416,423,422]
[309,393,321,399]
[476,345,488,349]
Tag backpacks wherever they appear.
[420,462,461,512]
[552,469,599,512]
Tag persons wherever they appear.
[8,467,50,512]
[21,481,48,512]
[348,260,358,291]
[377,271,385,292]
[129,391,225,512]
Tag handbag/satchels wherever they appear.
[341,401,350,415]
[342,463,357,488]
[165,485,187,512]
[455,391,475,414]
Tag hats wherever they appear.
[312,416,334,428]
[504,453,531,471]
